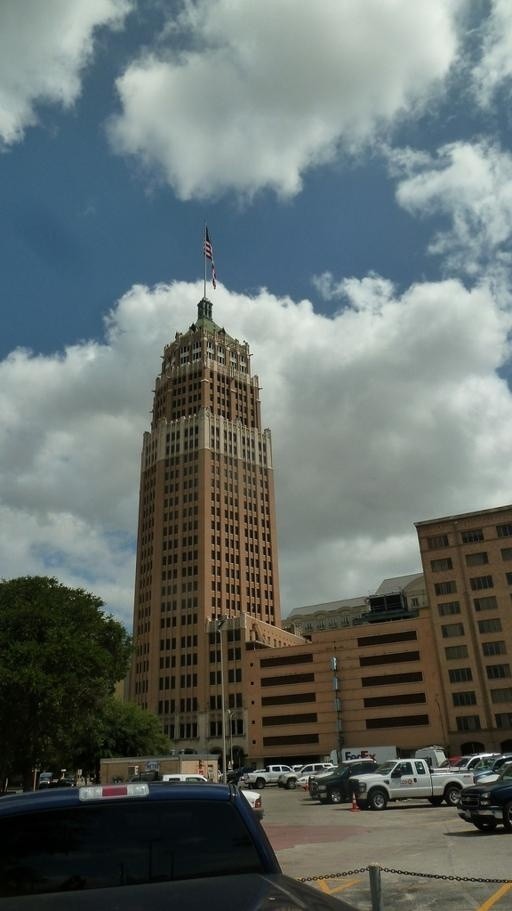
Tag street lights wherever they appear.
[225,709,239,773]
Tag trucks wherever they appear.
[329,746,448,770]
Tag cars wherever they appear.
[2,782,359,911]
[296,767,336,791]
[440,751,512,787]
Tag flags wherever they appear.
[203,227,217,291]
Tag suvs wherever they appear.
[457,764,512,833]
[279,763,334,791]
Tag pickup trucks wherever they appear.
[309,761,381,806]
[348,760,475,811]
[243,765,295,790]
[162,773,265,822]
